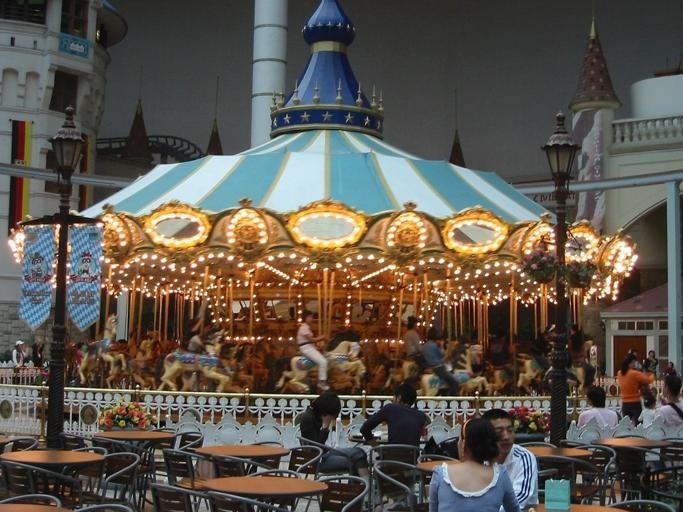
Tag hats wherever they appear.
[16,340,24,345]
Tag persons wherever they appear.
[10,325,163,384]
[577,349,683,495]
[401,316,596,395]
[202,319,222,342]
[296,311,332,392]
[299,392,381,504]
[430,409,539,512]
[180,298,211,357]
[360,383,432,508]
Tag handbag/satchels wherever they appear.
[544,478,571,510]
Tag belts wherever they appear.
[298,342,314,347]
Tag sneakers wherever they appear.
[317,382,330,391]
[363,496,392,511]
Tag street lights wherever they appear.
[46,104,87,450]
[539,110,582,442]
